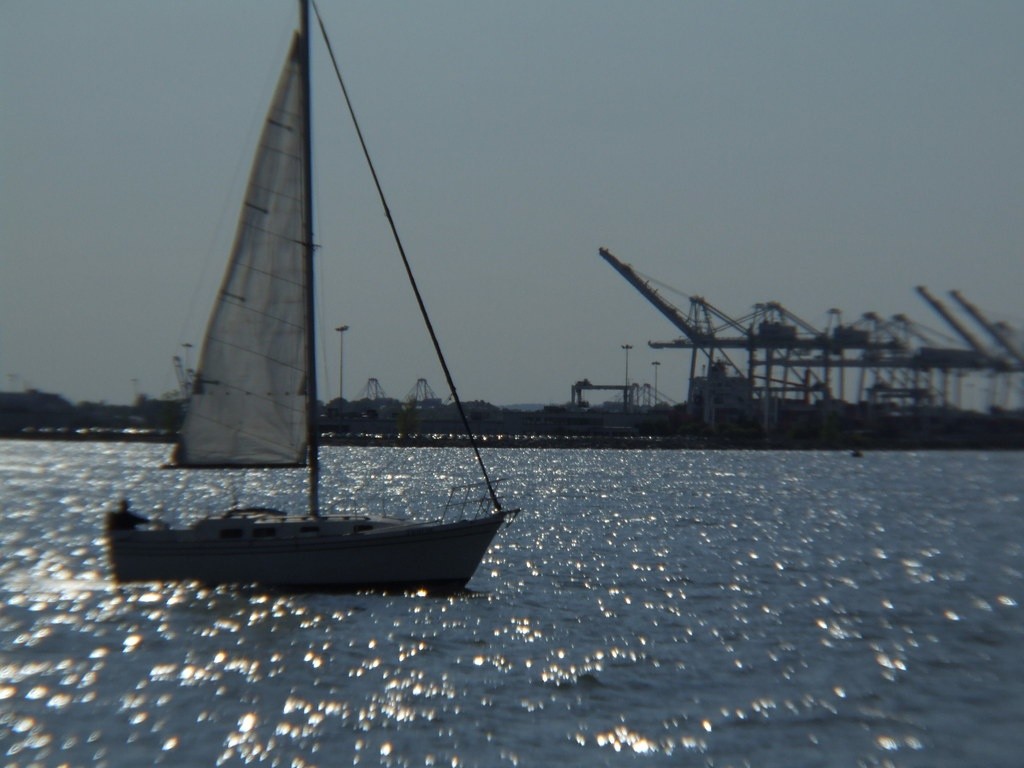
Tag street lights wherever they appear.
[334,325,349,438]
[620,343,633,434]
[652,361,660,440]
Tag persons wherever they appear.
[106,499,159,530]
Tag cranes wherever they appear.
[596,245,1024,451]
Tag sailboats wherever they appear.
[108,0,525,594]
